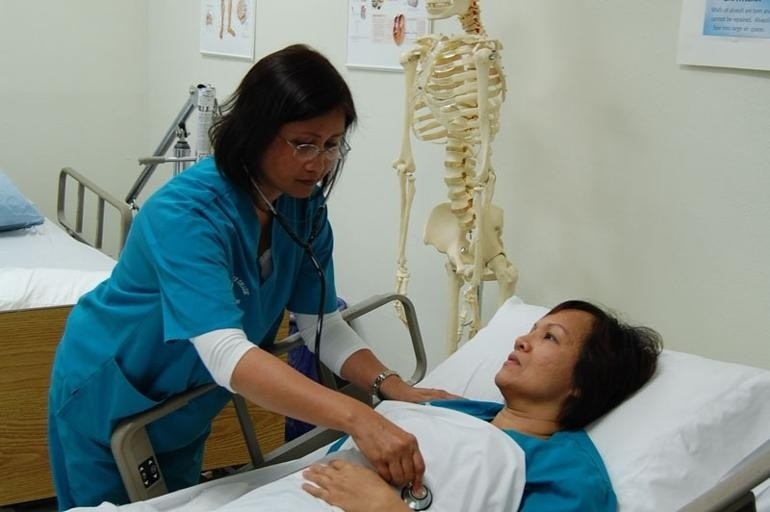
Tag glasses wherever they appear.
[278,129,351,162]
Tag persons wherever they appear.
[44,41,473,512]
[215,295,665,512]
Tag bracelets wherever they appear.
[371,369,404,400]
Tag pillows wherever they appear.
[0,170,44,233]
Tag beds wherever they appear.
[0,168,131,508]
[54,292,768,511]
